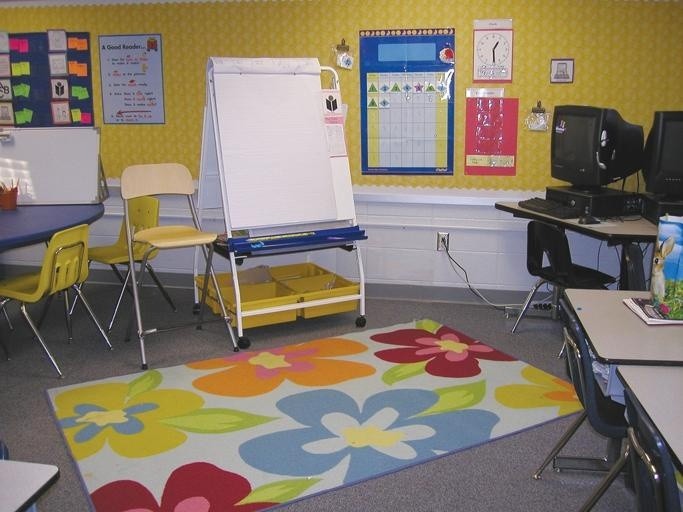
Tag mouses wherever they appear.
[574,212,600,224]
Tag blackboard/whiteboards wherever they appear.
[0,127,102,206]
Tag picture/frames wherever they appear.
[98,33,165,124]
[550,59,575,83]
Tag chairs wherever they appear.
[119,162,239,370]
[578,386,682,512]
[530,297,630,479]
[0,223,113,379]
[512,220,617,360]
[68,198,178,334]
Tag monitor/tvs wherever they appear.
[543,105,646,217]
[642,109,683,223]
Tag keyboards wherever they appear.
[521,197,575,220]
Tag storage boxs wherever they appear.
[196,263,362,328]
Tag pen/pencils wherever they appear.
[0,178,20,192]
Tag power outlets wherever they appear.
[437,232,449,252]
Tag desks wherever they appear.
[616,365,682,477]
[0,460,61,512]
[553,288,683,473]
[1,207,104,360]
[494,201,659,291]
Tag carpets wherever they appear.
[46,316,587,512]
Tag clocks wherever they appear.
[472,28,514,83]
